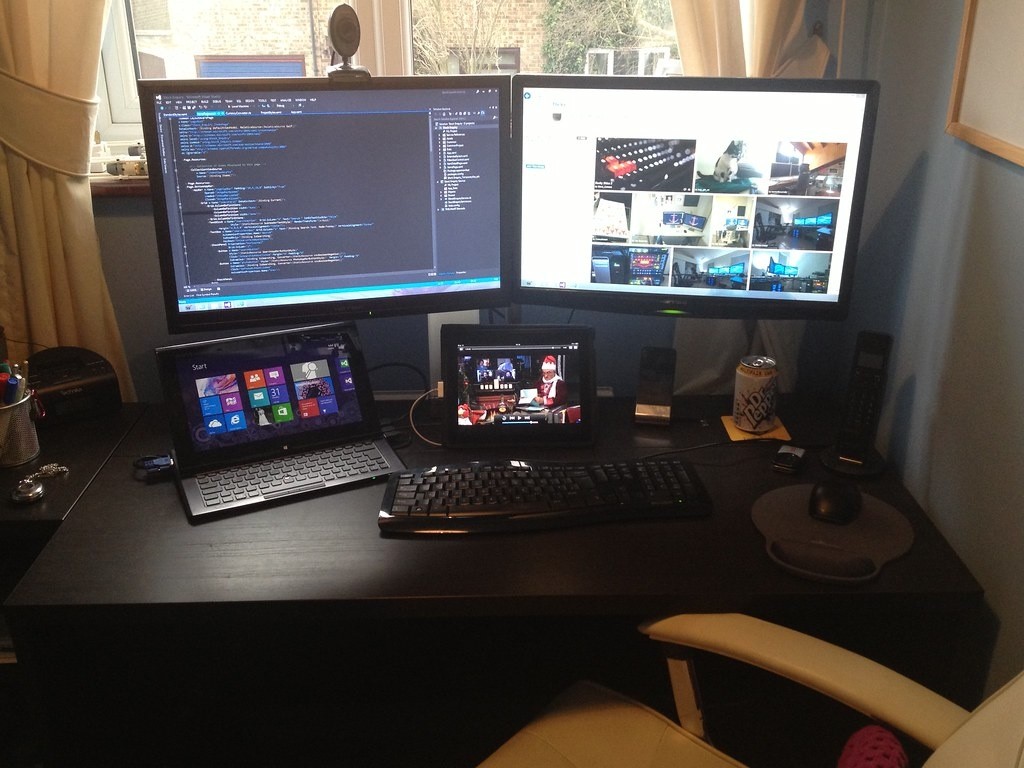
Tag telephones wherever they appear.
[819,330,895,478]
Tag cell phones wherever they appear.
[773,445,805,474]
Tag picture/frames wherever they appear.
[942,0,1024,170]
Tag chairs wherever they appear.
[475,598,1024,768]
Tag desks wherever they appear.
[1,398,149,541]
[1,397,987,768]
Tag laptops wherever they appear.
[154,319,407,526]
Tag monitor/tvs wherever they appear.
[511,75,881,320]
[440,324,596,447]
[136,75,512,336]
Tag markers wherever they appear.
[0,357,29,458]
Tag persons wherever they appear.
[531,353,569,408]
[505,370,514,381]
[480,371,493,382]
[477,359,485,367]
[251,407,273,426]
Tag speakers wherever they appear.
[634,347,678,424]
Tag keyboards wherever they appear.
[378,454,714,539]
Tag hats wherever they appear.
[541,355,556,371]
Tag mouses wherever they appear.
[808,480,864,525]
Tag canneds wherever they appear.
[733,355,777,434]
